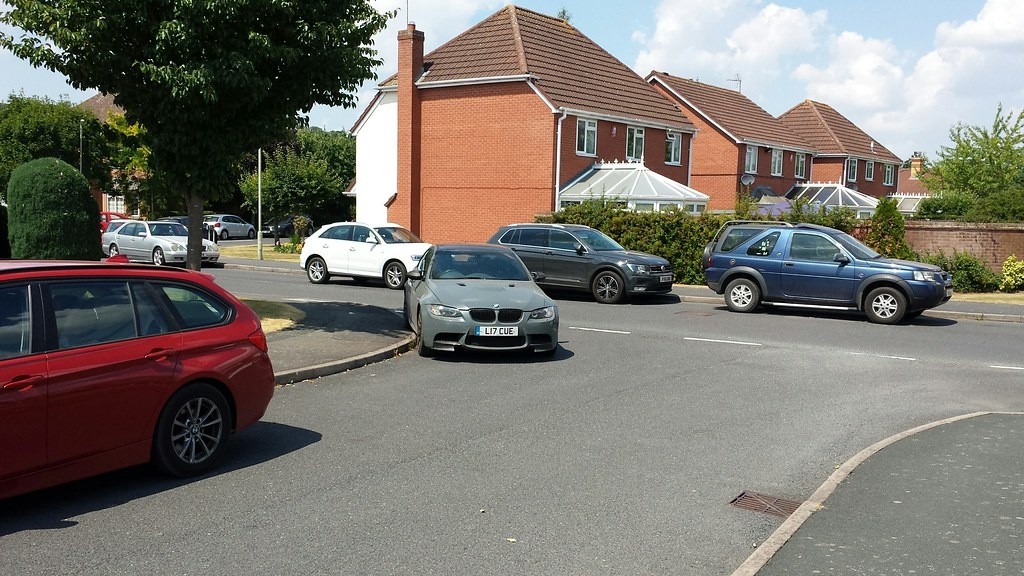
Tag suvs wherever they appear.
[469,222,675,304]
[701,220,953,325]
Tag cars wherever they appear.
[298,223,456,290]
[102,221,220,267]
[261,214,314,238]
[99,211,134,232]
[150,216,205,238]
[203,214,257,241]
[403,244,559,357]
[0,255,276,505]
